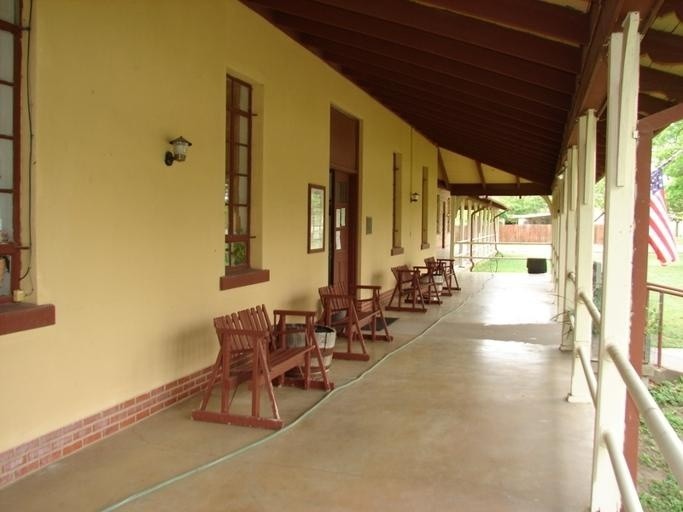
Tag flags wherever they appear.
[647,170,676,269]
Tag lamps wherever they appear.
[165,136,192,166]
[411,192,420,201]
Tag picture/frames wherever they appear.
[308,184,326,253]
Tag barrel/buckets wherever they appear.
[420,273,444,300]
[277,323,336,377]
